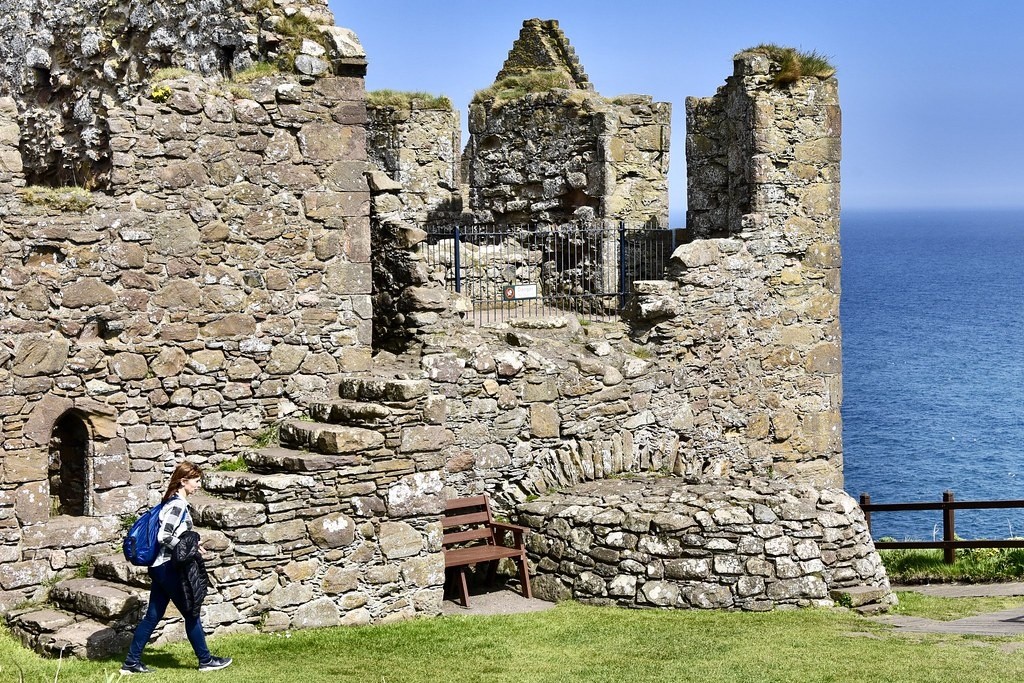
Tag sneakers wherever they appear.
[118,661,149,675]
[199,655,233,671]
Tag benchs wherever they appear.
[442,494,533,607]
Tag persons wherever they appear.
[121,461,233,674]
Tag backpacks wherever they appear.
[122,498,186,566]
